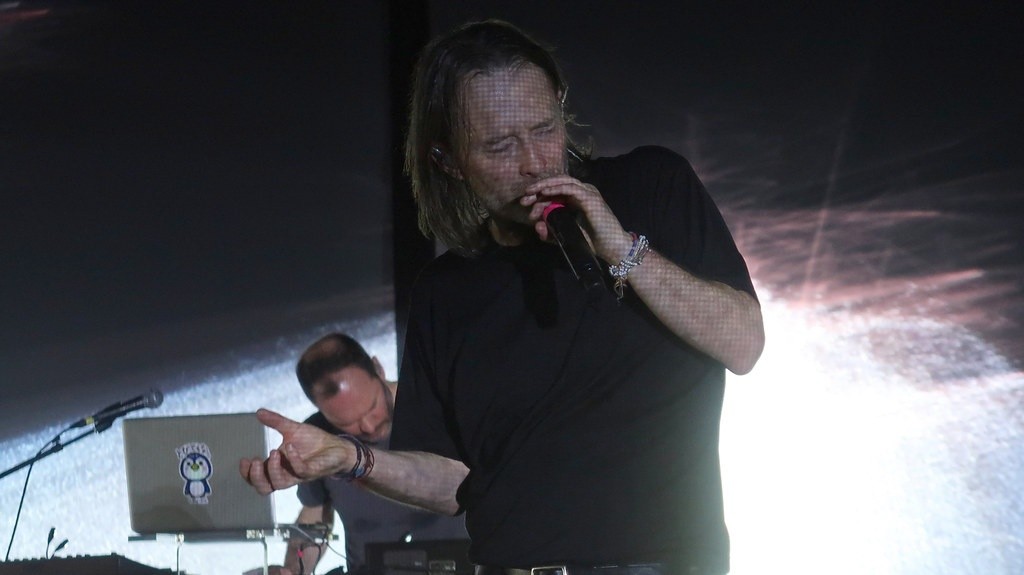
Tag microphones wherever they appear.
[71,388,164,430]
[536,192,606,297]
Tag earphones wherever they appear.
[435,150,451,169]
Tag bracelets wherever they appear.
[609,231,650,294]
[331,435,374,484]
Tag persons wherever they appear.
[242,331,470,575]
[240,21,765,575]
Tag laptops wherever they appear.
[121,411,277,535]
